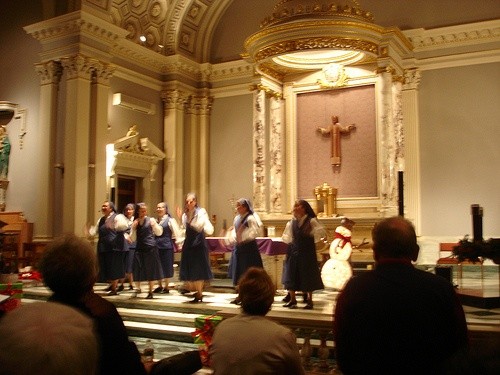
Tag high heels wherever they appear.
[189,296,203,303]
[180,288,189,294]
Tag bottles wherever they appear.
[143,339,154,362]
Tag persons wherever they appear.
[316,116,356,167]
[331,216,472,375]
[1,193,326,375]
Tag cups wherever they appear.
[267,227,276,237]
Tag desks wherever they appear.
[174,237,288,296]
[260,215,355,250]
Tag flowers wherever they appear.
[449,237,478,263]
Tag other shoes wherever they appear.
[152,287,162,293]
[283,300,297,308]
[107,291,117,295]
[128,285,133,290]
[145,292,153,299]
[117,285,124,291]
[302,302,313,309]
[160,288,169,294]
[106,286,112,291]
[282,293,290,302]
[231,299,241,305]
[303,294,307,303]
